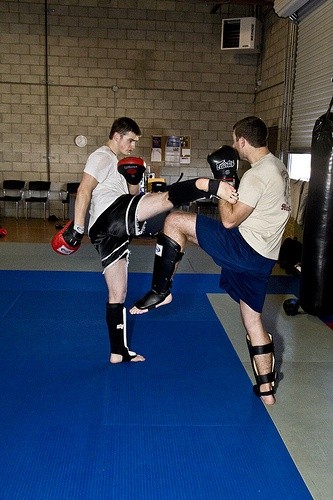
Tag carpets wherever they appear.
[0,269,314,500]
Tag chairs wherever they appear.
[24,180,52,221]
[59,182,81,223]
[0,179,27,221]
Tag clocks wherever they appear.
[74,134,88,148]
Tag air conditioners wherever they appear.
[219,16,264,55]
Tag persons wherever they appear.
[51,117,238,363]
[129,115,291,404]
